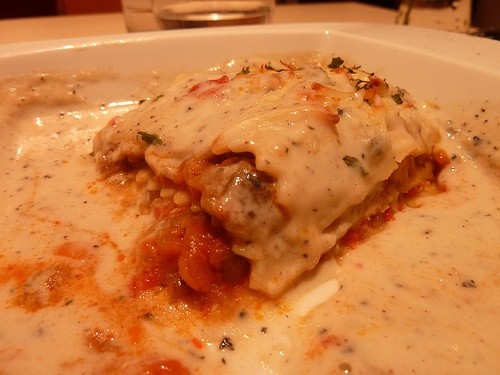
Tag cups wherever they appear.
[121,0,276,33]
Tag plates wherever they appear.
[0,22,499,375]
